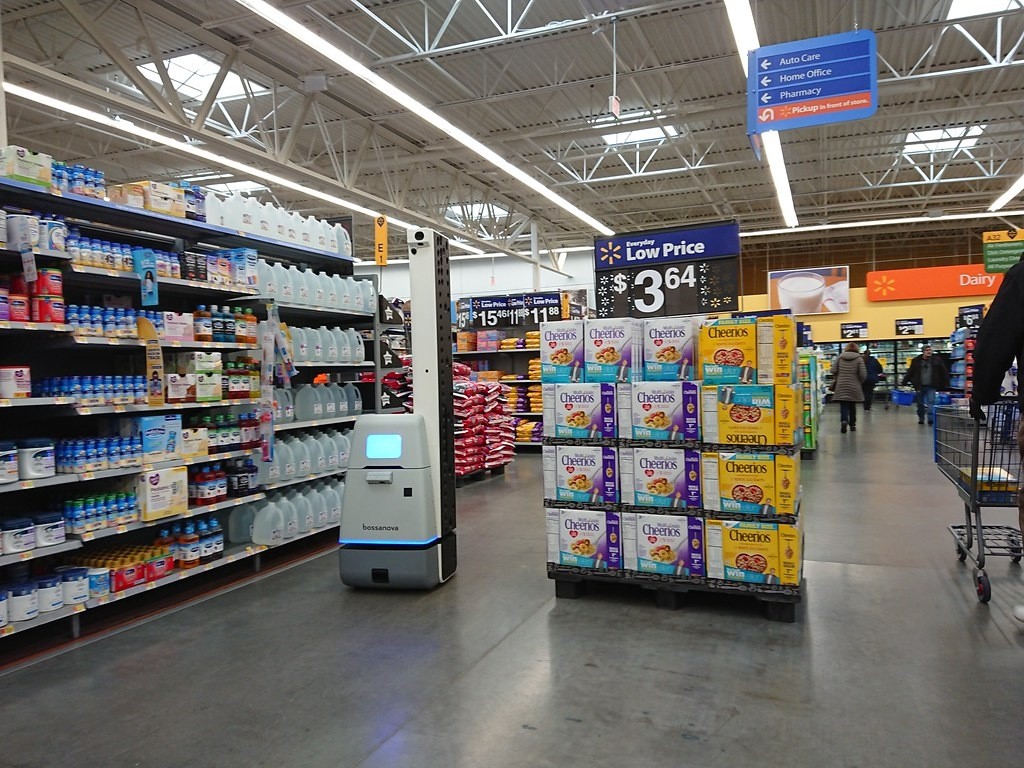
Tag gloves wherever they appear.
[969,398,986,421]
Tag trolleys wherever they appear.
[938,388,1023,601]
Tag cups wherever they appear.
[775,272,825,316]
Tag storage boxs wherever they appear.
[121,414,182,465]
[164,311,193,341]
[107,180,186,218]
[892,389,917,406]
[164,352,223,404]
[0,145,52,187]
[960,467,1021,503]
[477,370,505,382]
[539,314,805,587]
[182,428,208,459]
[456,330,506,352]
[118,466,188,521]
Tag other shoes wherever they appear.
[1013,605,1024,622]
[865,408,873,412]
[919,421,924,424]
[928,420,933,424]
[850,427,856,431]
[841,421,848,433]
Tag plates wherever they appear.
[823,280,848,312]
[644,413,672,431]
[566,473,593,493]
[594,349,622,365]
[566,412,591,428]
[646,481,674,497]
[569,540,596,558]
[549,350,574,366]
[656,348,682,366]
[650,547,677,564]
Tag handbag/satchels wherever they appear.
[828,359,839,391]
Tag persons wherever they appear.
[902,343,951,424]
[858,349,883,412]
[616,360,630,383]
[966,252,1024,625]
[569,360,581,382]
[676,358,690,381]
[590,488,599,503]
[764,568,777,584]
[668,424,680,441]
[761,498,772,517]
[720,386,736,411]
[670,492,683,507]
[831,342,868,433]
[588,423,597,439]
[592,553,603,569]
[673,559,685,575]
[738,361,754,384]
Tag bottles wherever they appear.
[52,159,376,592]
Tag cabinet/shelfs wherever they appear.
[70,194,269,639]
[897,336,954,386]
[840,339,897,389]
[0,174,86,639]
[949,342,965,389]
[452,348,543,445]
[256,236,380,573]
[815,342,840,376]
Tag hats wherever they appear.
[845,343,859,351]
[921,344,930,350]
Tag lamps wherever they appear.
[305,71,336,94]
[723,0,800,228]
